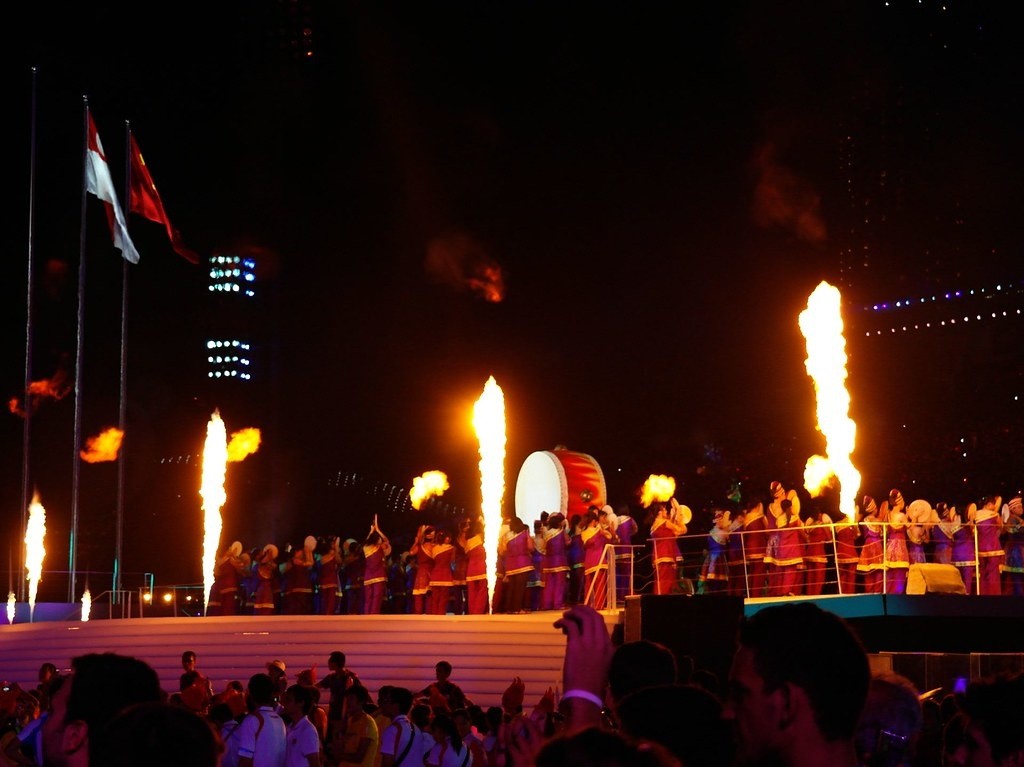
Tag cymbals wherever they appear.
[670,504,692,525]
[879,500,889,519]
[787,489,800,515]
[264,544,278,559]
[304,536,317,550]
[230,541,243,558]
[1002,503,1010,524]
[993,495,1001,511]
[907,500,931,522]
[964,503,977,522]
[602,504,618,532]
[344,539,357,551]
[805,513,833,527]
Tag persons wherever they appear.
[0,483,1024,767]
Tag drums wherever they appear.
[515,444,606,538]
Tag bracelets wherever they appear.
[560,688,603,709]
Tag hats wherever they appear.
[295,670,311,682]
[889,488,903,506]
[937,502,949,519]
[770,481,784,498]
[712,510,731,523]
[266,660,285,673]
[863,496,876,513]
[1008,497,1022,510]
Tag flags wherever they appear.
[87,107,141,266]
[129,131,202,265]
